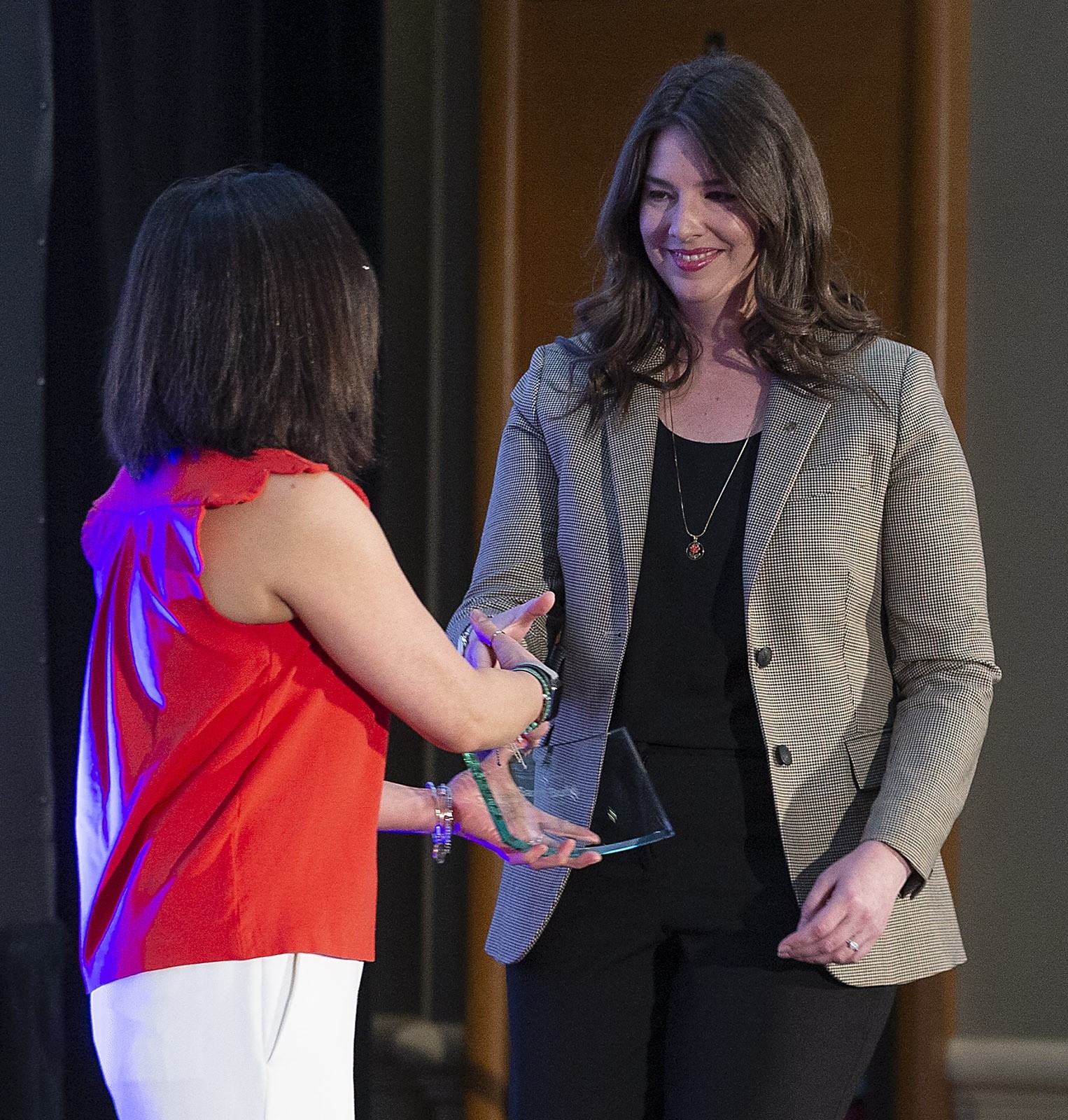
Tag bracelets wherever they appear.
[496,662,552,769]
[424,780,453,863]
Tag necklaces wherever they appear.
[664,350,772,561]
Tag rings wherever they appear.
[488,630,506,653]
[845,939,859,952]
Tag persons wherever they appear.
[75,166,601,1120]
[446,52,1002,1120]
